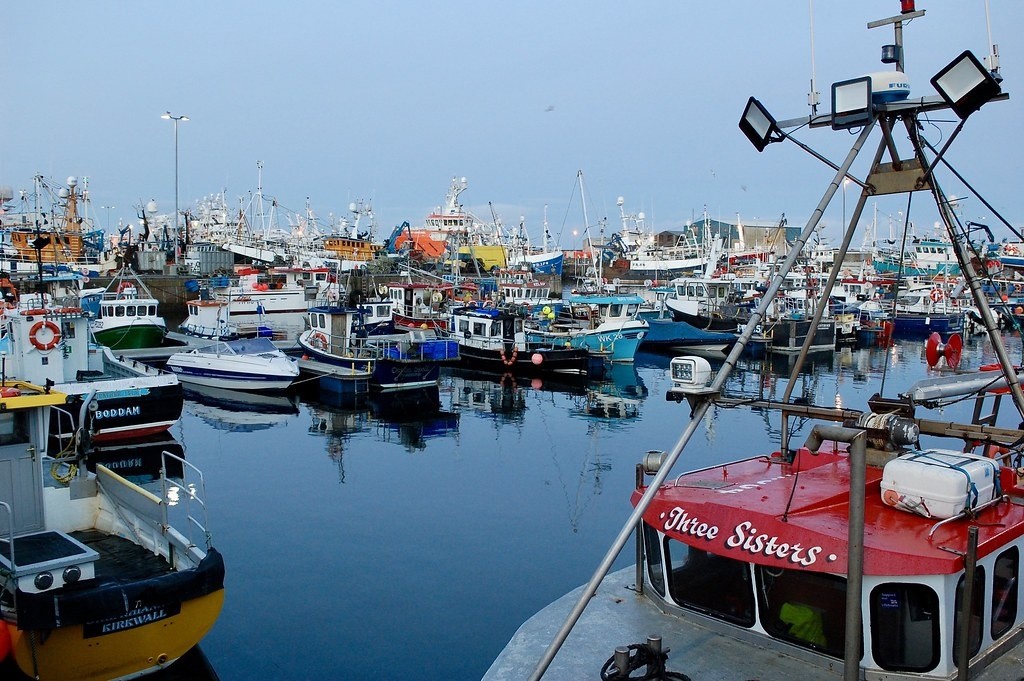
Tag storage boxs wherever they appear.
[880,449,1002,521]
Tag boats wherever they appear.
[0,0,1024,681]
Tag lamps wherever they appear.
[930,50,1002,121]
[666,355,712,401]
[738,96,785,152]
[830,76,873,131]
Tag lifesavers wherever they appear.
[53,308,81,313]
[465,301,478,307]
[311,333,327,351]
[29,321,61,350]
[929,288,943,302]
[19,309,52,315]
[482,300,495,308]
[118,282,134,300]
[988,444,1013,469]
